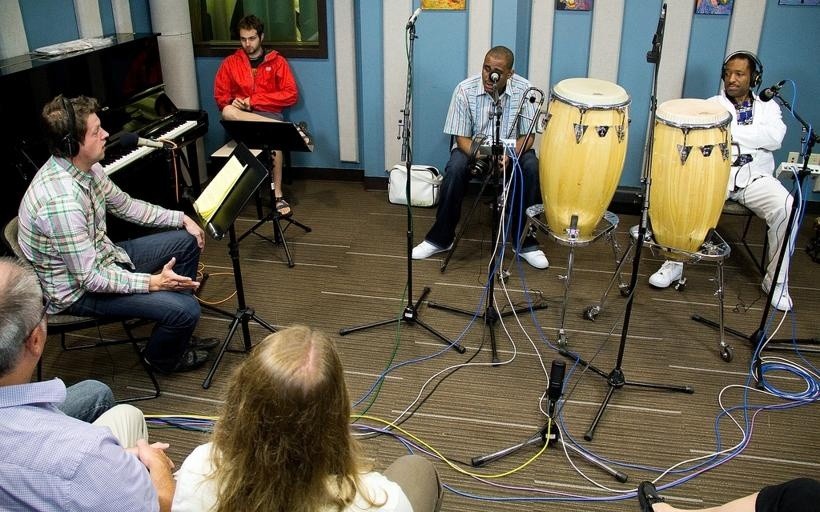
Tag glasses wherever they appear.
[24,293,51,344]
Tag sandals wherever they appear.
[298,120,313,145]
[274,195,294,217]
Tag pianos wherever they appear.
[1,32,208,258]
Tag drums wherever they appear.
[652,98,732,262]
[539,78,631,246]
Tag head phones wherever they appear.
[721,50,763,86]
[57,96,80,157]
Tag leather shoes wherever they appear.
[184,336,220,349]
[638,482,665,511]
[142,350,209,373]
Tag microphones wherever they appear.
[404,8,424,30]
[546,359,566,403]
[759,79,787,102]
[119,131,167,152]
[489,71,501,84]
[647,3,667,63]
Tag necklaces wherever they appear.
[246,51,263,60]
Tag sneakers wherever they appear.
[512,248,550,269]
[412,240,453,260]
[649,261,684,288]
[761,273,793,311]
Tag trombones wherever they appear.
[439,88,545,275]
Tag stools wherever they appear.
[723,200,765,277]
[440,133,517,272]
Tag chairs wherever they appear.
[4,216,161,405]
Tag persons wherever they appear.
[213,13,300,217]
[171,320,444,512]
[18,91,220,376]
[1,255,177,511]
[649,50,794,312]
[638,476,820,511]
[48,377,114,425]
[408,45,552,272]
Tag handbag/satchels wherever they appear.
[388,164,444,208]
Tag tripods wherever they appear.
[199,226,282,389]
[229,150,311,267]
[692,100,820,390]
[426,89,548,367]
[471,403,628,483]
[559,45,694,441]
[339,27,466,355]
